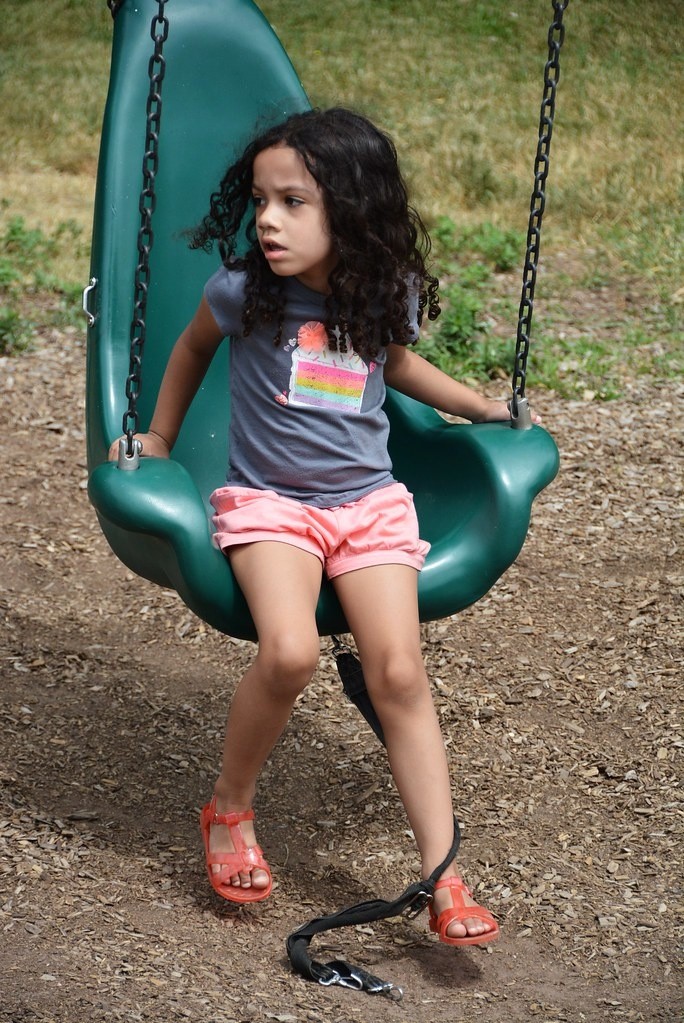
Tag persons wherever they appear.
[103,102,546,949]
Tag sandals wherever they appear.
[200,795,273,903]
[427,875,500,946]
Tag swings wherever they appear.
[84,0,572,641]
